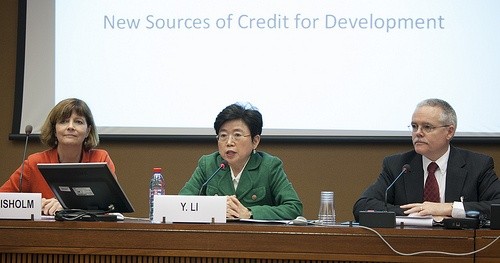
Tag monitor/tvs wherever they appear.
[36,162,135,222]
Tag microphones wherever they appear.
[359,164,412,228]
[19,125,33,193]
[199,160,228,195]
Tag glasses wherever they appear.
[407,124,452,133]
[216,133,253,142]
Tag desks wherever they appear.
[0,217,500,263]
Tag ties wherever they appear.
[423,163,441,203]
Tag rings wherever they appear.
[420,206,423,210]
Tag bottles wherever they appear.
[149,168,166,222]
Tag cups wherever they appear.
[318,191,336,225]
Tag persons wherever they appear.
[352,99,500,223]
[177,101,303,220]
[0,98,115,216]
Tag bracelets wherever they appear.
[247,208,253,219]
